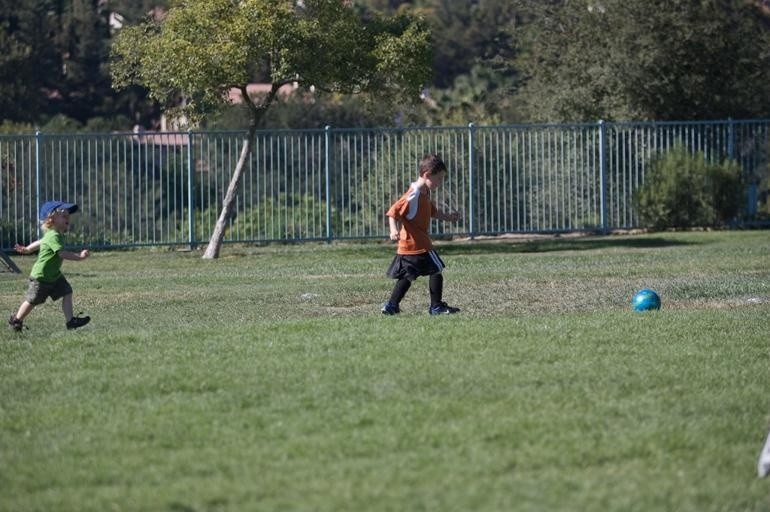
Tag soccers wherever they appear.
[632,289,660,312]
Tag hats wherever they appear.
[40,201,79,221]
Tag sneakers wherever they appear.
[8,315,29,333]
[66,311,91,330]
[380,302,403,316]
[429,301,460,316]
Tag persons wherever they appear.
[8,200,91,333]
[383,156,461,315]
